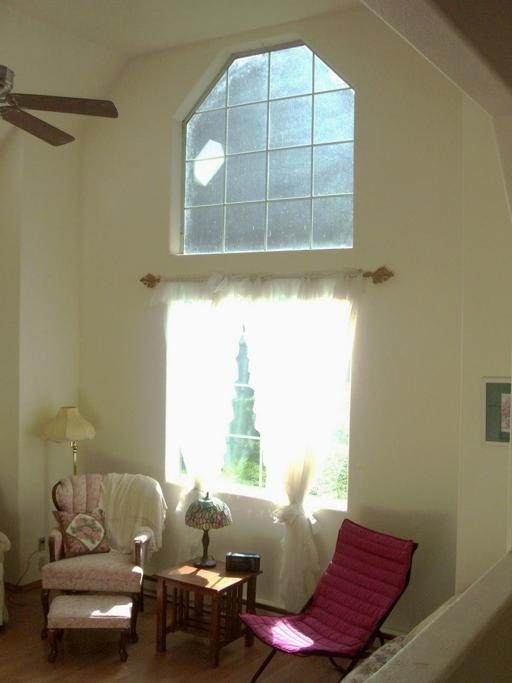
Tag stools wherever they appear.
[44,594,134,663]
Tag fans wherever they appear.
[0,65,119,148]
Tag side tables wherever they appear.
[153,555,263,664]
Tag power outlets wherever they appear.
[37,535,49,571]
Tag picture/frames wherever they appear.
[483,380,511,446]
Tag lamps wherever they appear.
[41,406,95,476]
[185,491,233,567]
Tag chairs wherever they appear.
[41,473,169,644]
[239,518,419,683]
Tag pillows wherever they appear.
[51,508,111,558]
